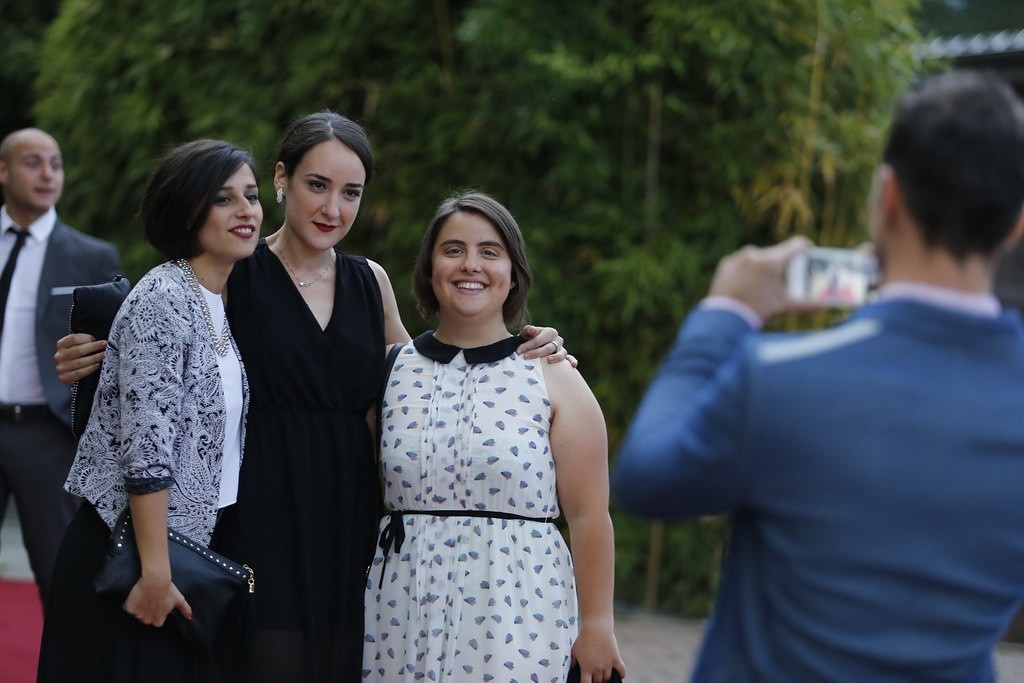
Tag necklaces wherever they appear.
[277,230,335,287]
[176,258,230,357]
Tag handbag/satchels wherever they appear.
[94,502,254,655]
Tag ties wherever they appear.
[0,228,30,341]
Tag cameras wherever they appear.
[784,244,878,310]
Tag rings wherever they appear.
[550,340,558,350]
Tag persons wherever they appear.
[0,128,124,619]
[52,113,578,683]
[36,139,263,683]
[362,193,626,683]
[613,71,1024,683]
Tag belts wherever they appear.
[0,404,56,423]
